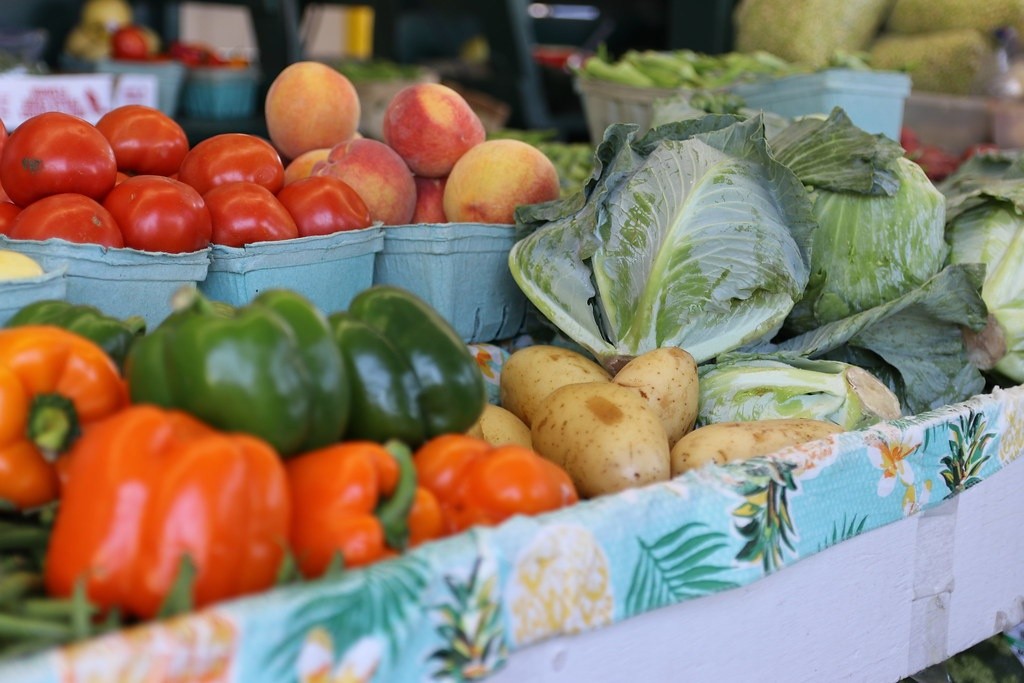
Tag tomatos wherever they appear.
[0,105,375,253]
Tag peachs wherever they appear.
[263,60,559,226]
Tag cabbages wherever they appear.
[508,104,1024,433]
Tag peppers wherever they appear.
[0,287,578,623]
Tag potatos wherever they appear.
[461,345,840,498]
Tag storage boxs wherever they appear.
[0,0,1024,683]
